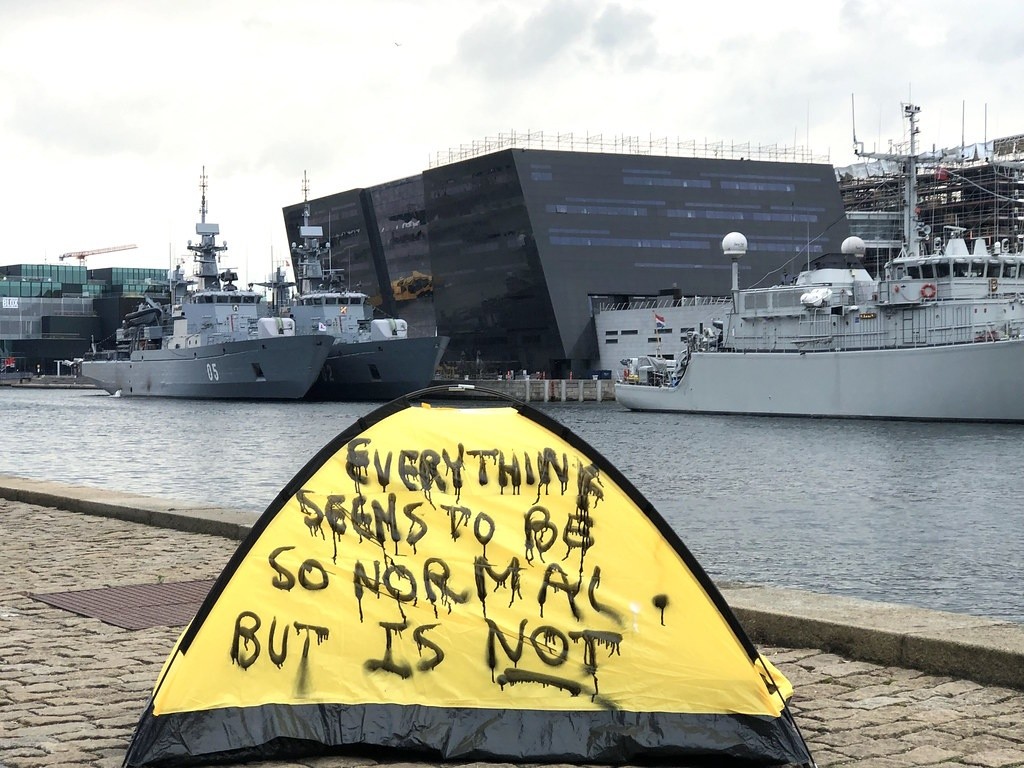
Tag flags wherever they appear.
[655,315,665,328]
[936,171,947,179]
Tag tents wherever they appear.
[121,384,818,768]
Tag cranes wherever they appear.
[58,244,138,264]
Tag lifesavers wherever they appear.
[989,278,998,293]
[623,368,628,380]
[921,284,936,300]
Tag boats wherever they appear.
[74,165,337,400]
[125,309,161,326]
[259,167,449,403]
[612,96,1023,423]
